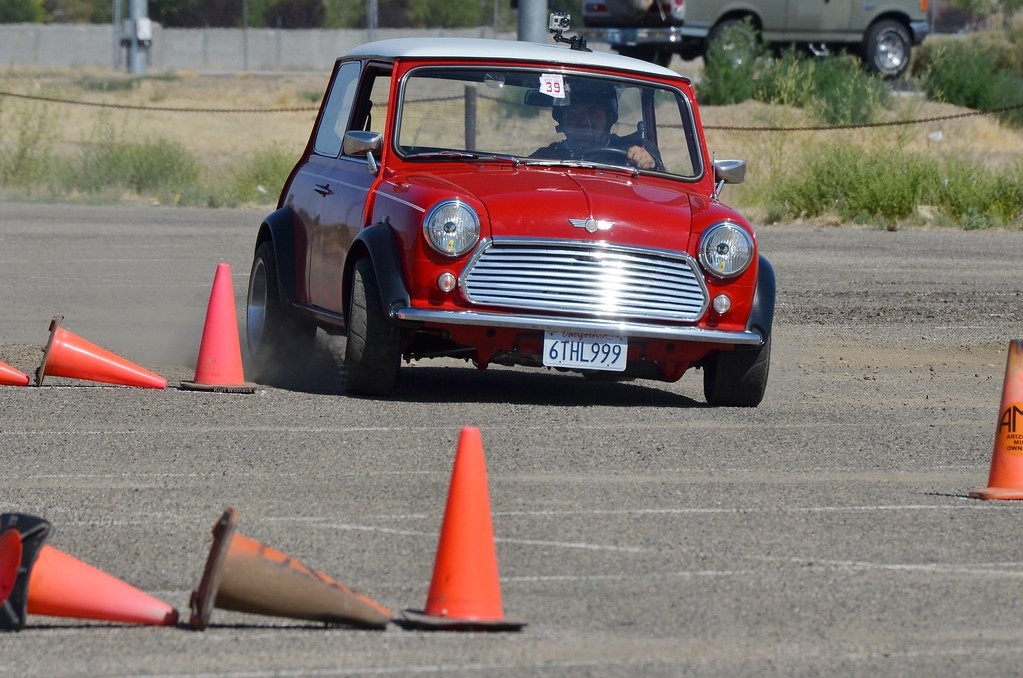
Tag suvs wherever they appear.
[548,0,932,105]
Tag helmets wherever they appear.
[552,76,619,124]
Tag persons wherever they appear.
[530,77,666,171]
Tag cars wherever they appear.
[244,36,774,409]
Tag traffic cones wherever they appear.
[179,260,256,393]
[34,316,168,390]
[970,341,1023,502]
[0,512,179,632]
[399,426,527,629]
[189,505,391,630]
[1,361,30,386]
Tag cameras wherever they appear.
[549,12,571,31]
